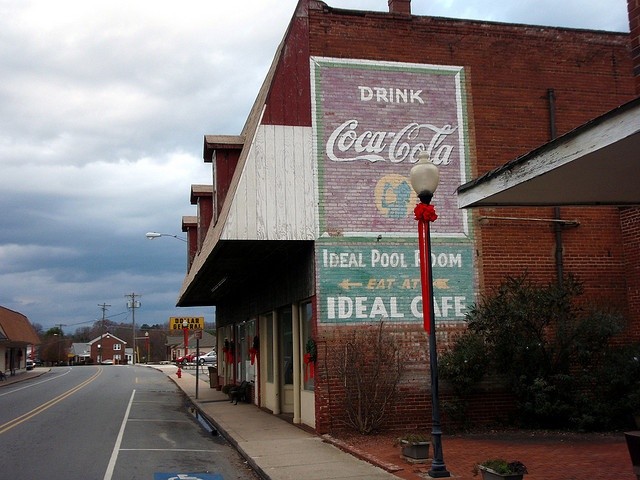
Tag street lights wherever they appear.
[405,149,451,478]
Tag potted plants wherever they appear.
[471,458,529,480]
[391,433,432,458]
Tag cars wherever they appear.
[175,351,205,363]
[193,352,220,365]
